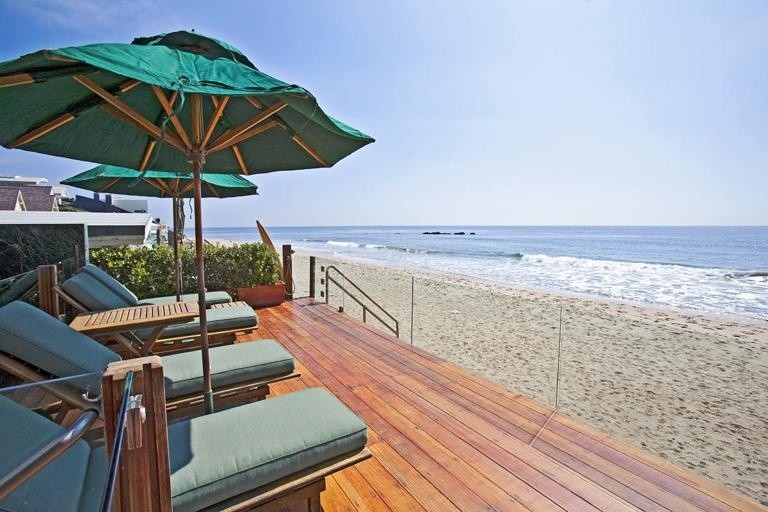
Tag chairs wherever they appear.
[1,298,302,440]
[0,387,375,512]
[51,271,262,359]
[75,262,234,311]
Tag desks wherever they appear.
[67,299,201,360]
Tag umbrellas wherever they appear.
[60,164,260,302]
[0,26,375,413]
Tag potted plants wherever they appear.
[235,242,286,308]
